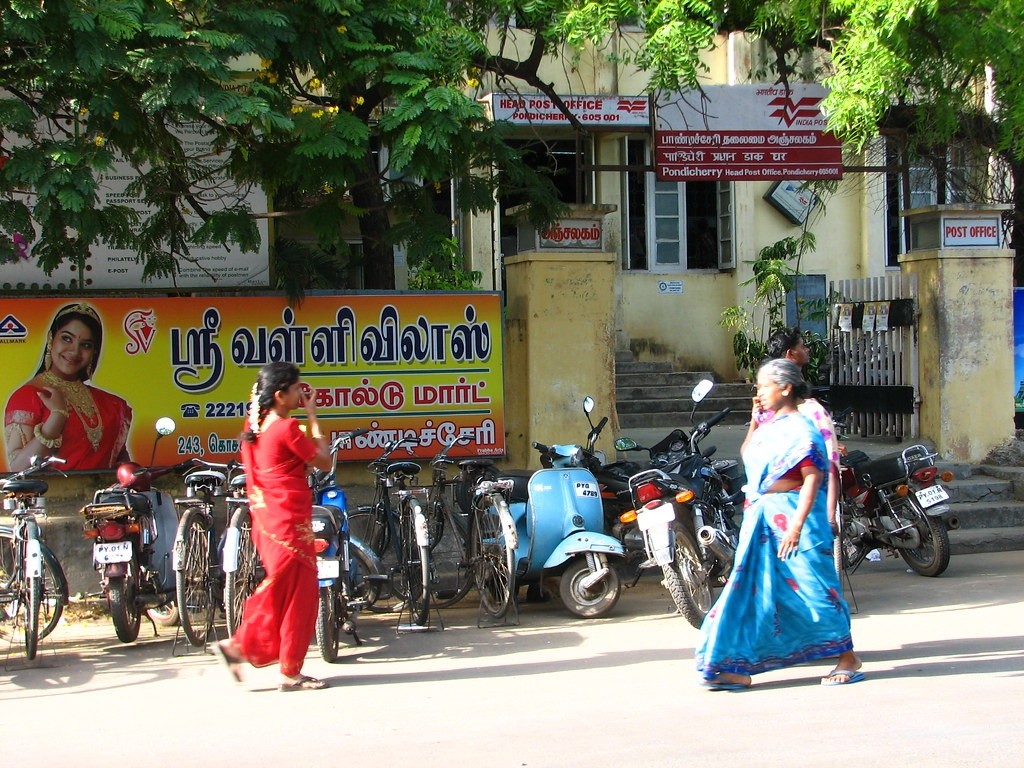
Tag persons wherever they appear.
[4,303,133,473]
[212,362,333,692]
[741,397,843,581]
[696,358,865,690]
[767,327,811,370]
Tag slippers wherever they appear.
[211,639,245,683]
[821,668,866,687]
[702,673,750,691]
[279,672,329,691]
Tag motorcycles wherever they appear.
[583,396,740,588]
[833,421,960,577]
[308,427,393,661]
[77,417,195,642]
[614,379,737,629]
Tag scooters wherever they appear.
[453,442,628,619]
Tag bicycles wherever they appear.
[172,456,245,656]
[222,460,267,641]
[348,437,445,636]
[388,434,521,629]
[1,454,69,673]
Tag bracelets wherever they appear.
[52,409,69,418]
[789,528,801,534]
[35,423,62,448]
